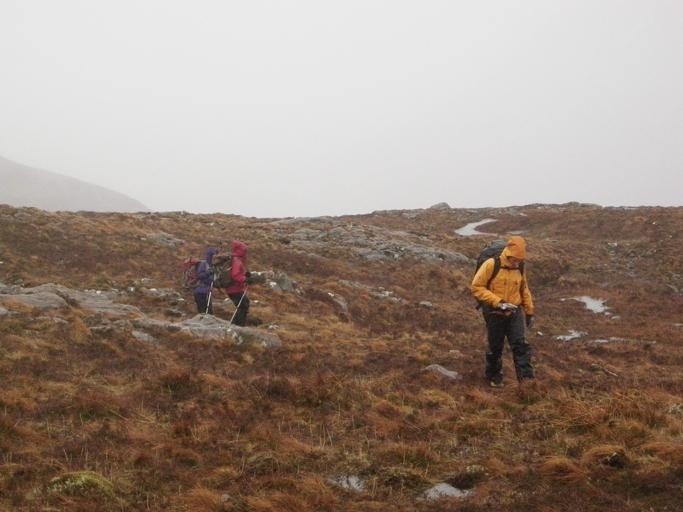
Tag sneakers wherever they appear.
[485,371,503,387]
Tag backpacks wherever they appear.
[474,239,525,279]
[211,254,231,289]
[182,256,200,290]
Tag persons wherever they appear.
[470,236,536,388]
[193,240,250,326]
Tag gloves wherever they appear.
[526,315,535,329]
[501,303,518,310]
